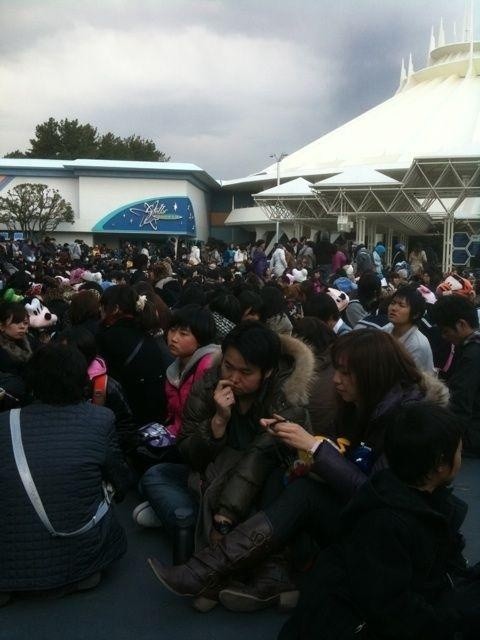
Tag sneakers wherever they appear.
[131,501,163,529]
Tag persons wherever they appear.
[0,228,480,639]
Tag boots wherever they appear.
[218,537,302,614]
[146,511,276,614]
